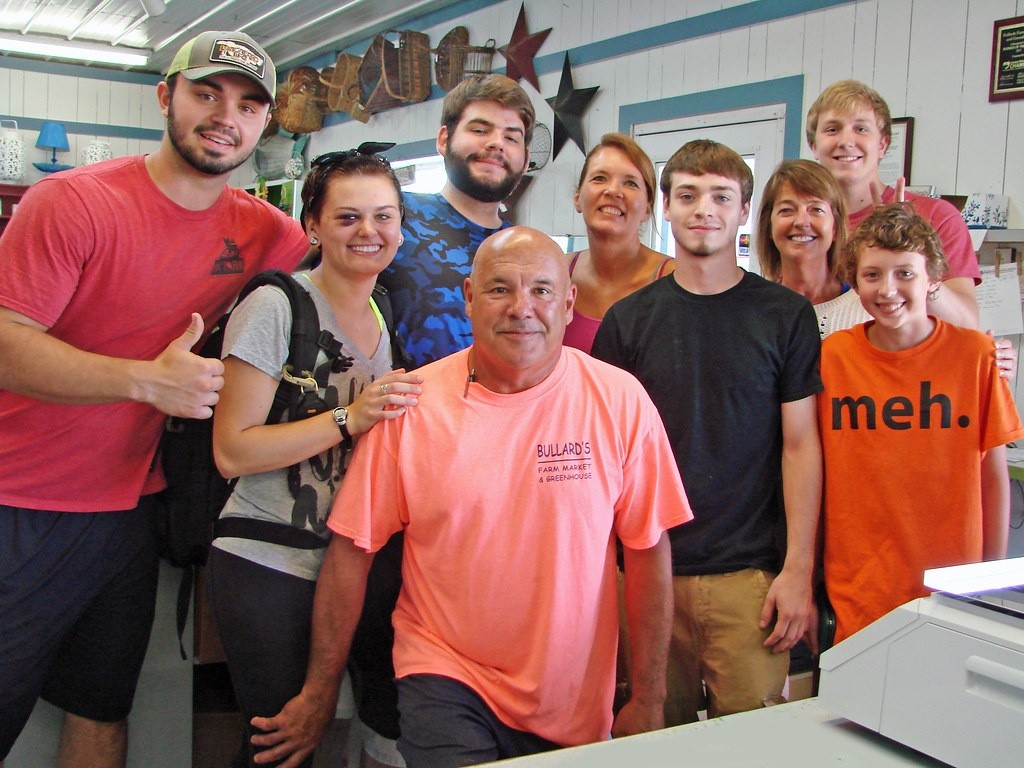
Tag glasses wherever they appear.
[307,150,406,224]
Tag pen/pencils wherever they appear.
[463,368,475,398]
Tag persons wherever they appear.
[307,75,536,372]
[0,31,312,768]
[589,138,825,724]
[250,227,697,768]
[803,201,1024,656]
[808,79,982,334]
[204,143,424,768]
[561,133,676,359]
[756,159,1016,382]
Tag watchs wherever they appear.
[330,407,354,452]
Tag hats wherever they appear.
[166,31,276,109]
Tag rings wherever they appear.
[380,384,388,395]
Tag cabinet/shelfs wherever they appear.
[243,178,305,223]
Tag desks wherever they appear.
[469,696,949,767]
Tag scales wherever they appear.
[816,557,1024,768]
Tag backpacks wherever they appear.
[142,268,395,665]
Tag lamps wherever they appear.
[141,0,167,18]
[32,121,75,172]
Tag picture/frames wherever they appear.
[877,117,914,187]
[987,16,1024,102]
